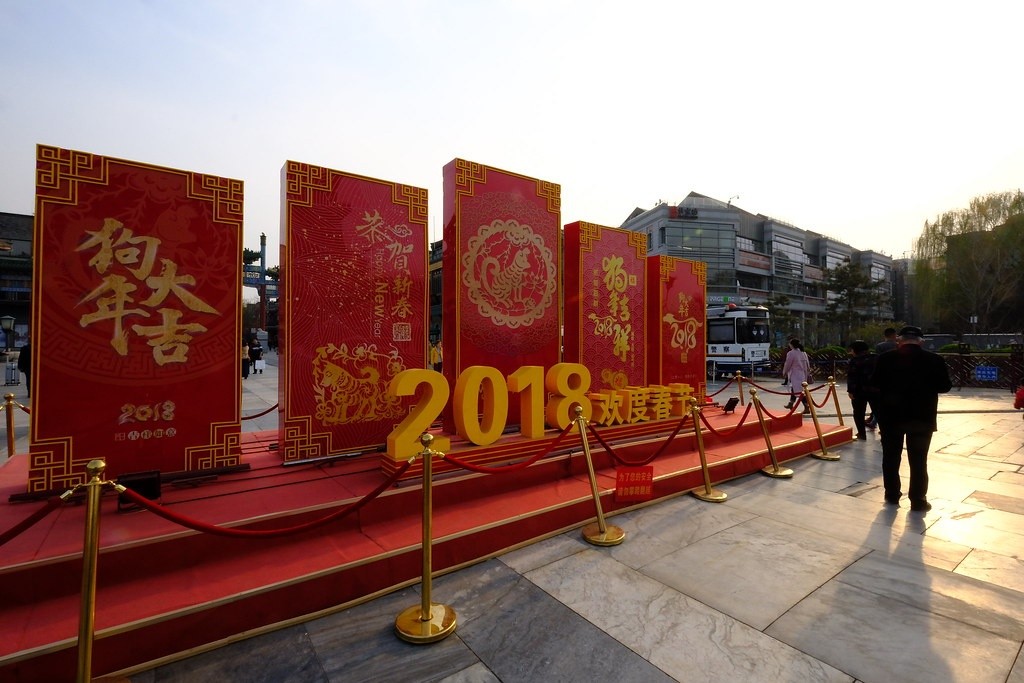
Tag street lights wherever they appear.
[1,315,16,352]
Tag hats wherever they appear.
[899,326,923,338]
[849,342,869,352]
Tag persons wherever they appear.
[781,338,812,414]
[847,326,951,510]
[18,335,31,397]
[242,338,264,380]
[428,340,442,373]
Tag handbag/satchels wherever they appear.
[807,374,814,384]
[255,360,265,369]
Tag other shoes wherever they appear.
[885,495,899,504]
[782,383,788,385]
[253,373,257,374]
[867,423,875,429]
[853,433,866,440]
[911,502,931,511]
[865,419,871,423]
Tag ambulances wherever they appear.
[706,303,771,380]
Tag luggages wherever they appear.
[4,356,20,386]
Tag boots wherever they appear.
[801,406,810,414]
[784,402,793,409]
